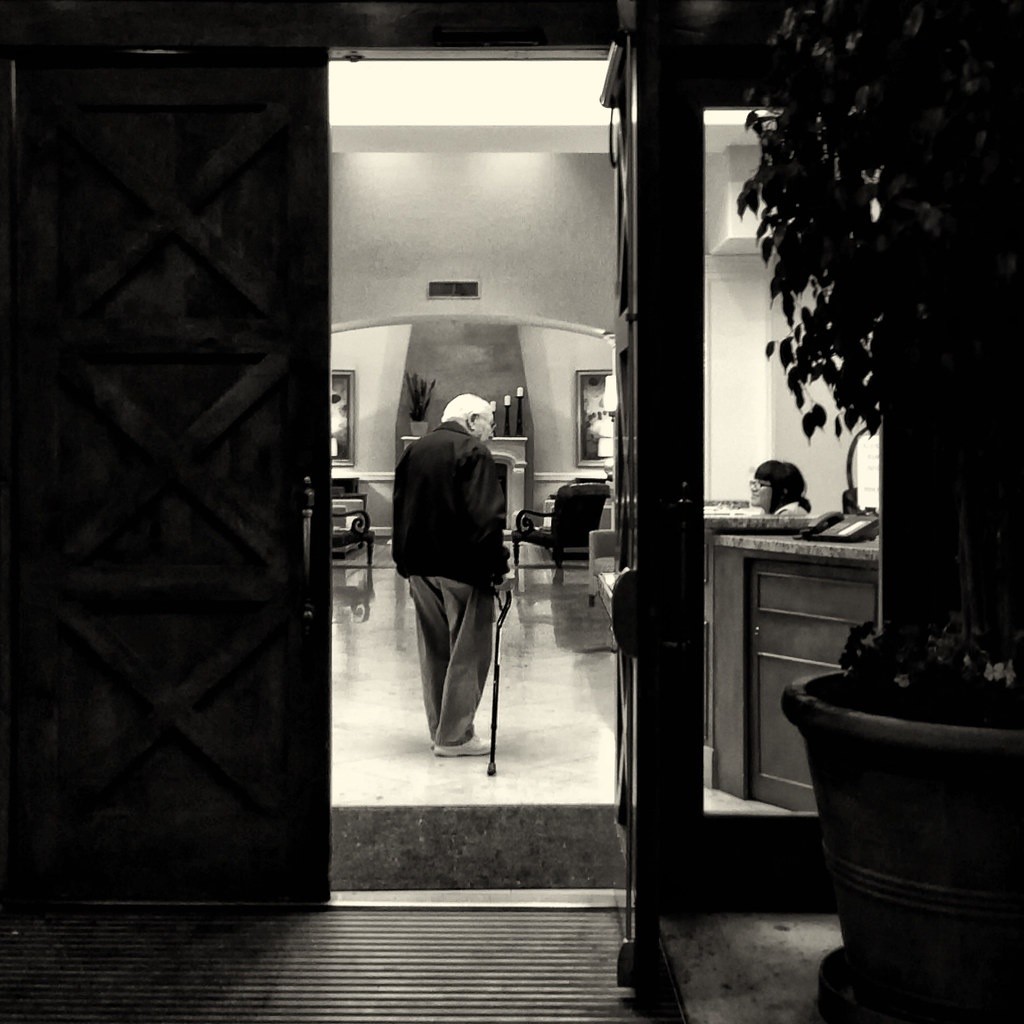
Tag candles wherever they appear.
[503,395,512,405]
[517,386,523,396]
[490,401,497,411]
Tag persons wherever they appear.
[393,393,515,757]
[750,460,811,516]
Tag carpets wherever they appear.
[329,805,628,891]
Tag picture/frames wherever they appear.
[576,370,613,467]
[332,370,356,467]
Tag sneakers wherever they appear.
[434,735,492,756]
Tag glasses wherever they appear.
[475,415,497,435]
[749,480,772,491]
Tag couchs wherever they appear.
[332,493,367,560]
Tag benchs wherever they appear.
[589,529,617,618]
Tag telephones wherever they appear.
[793,512,879,542]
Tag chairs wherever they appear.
[511,482,611,568]
[332,510,375,564]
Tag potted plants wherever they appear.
[406,372,436,435]
[781,619,1024,1024]
